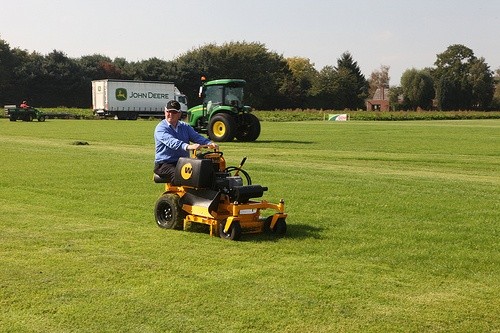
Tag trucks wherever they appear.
[90,79,188,121]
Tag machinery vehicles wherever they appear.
[151,144,288,242]
[186,78,261,142]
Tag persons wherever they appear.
[154,99,220,204]
[20,101,30,108]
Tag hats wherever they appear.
[165,100,181,112]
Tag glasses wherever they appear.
[166,111,180,114]
